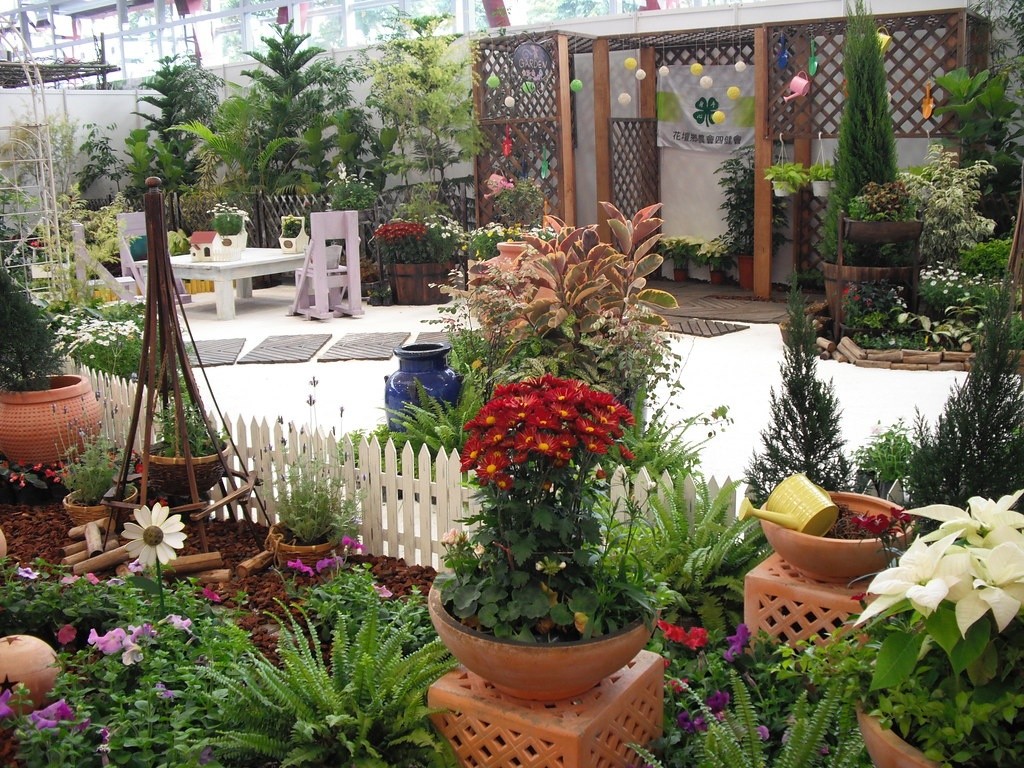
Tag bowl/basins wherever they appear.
[760,491,916,583]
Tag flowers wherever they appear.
[205,199,243,235]
[1,488,1024,768]
[845,179,918,222]
[280,213,301,237]
[251,377,369,547]
[324,160,380,208]
[916,258,1022,311]
[367,177,558,263]
[839,278,912,328]
[52,390,124,505]
[431,371,682,642]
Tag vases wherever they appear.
[268,520,345,578]
[843,217,924,243]
[760,494,916,586]
[840,322,917,340]
[384,341,460,433]
[386,261,455,305]
[428,567,659,702]
[856,699,945,766]
[63,484,139,527]
[478,241,558,336]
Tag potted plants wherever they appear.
[142,349,232,497]
[762,160,834,197]
[817,1,919,340]
[711,145,792,291]
[0,265,100,466]
[655,234,734,287]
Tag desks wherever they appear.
[125,246,312,321]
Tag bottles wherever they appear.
[384,342,461,431]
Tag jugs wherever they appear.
[783,71,810,102]
[877,27,892,54]
[739,475,838,532]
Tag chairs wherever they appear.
[115,212,192,305]
[288,211,365,322]
